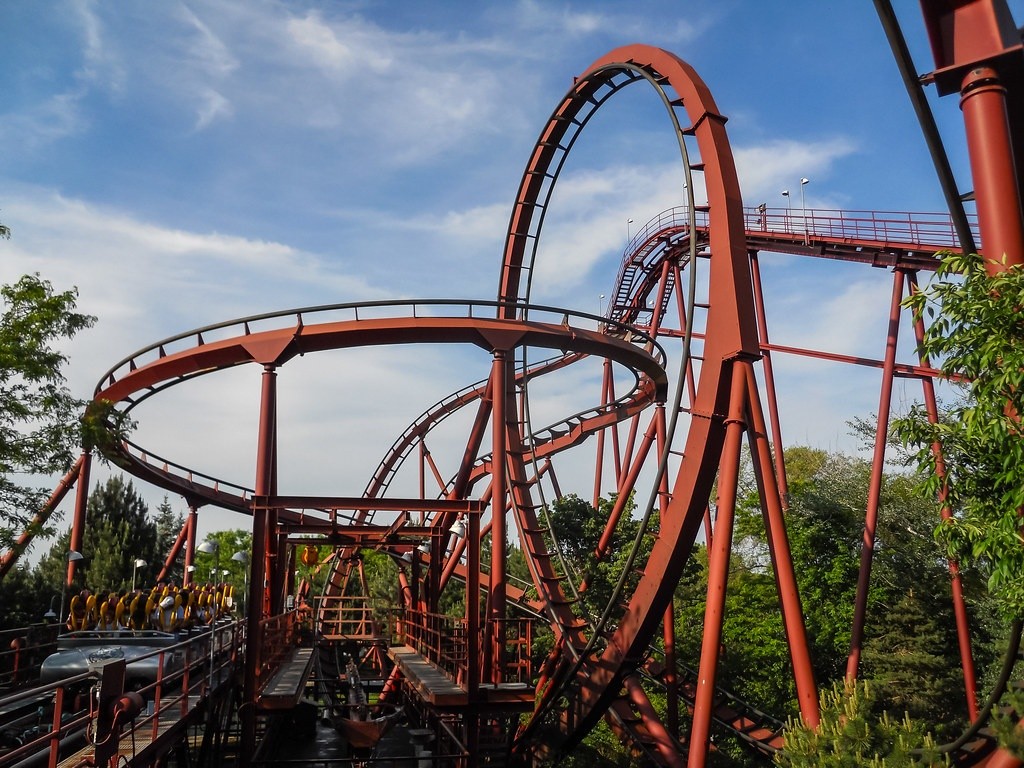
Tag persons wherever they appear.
[66,582,230,632]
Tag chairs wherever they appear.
[70,583,235,632]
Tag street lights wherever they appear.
[197,539,222,689]
[231,550,248,679]
[131,558,148,591]
[184,565,197,587]
[58,550,83,637]
